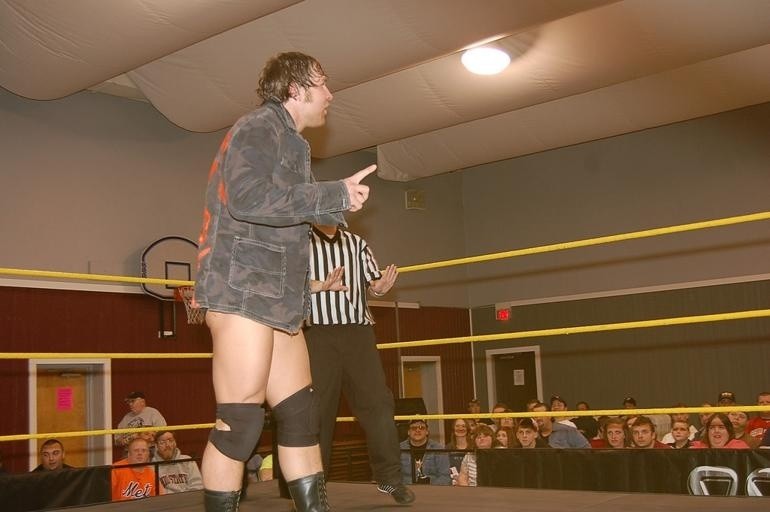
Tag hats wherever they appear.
[126,392,145,401]
[519,418,538,432]
[624,397,636,406]
[551,397,567,407]
[718,391,735,402]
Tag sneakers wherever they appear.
[377,479,415,504]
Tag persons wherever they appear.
[151,434,202,496]
[137,425,155,456]
[306,180,417,504]
[113,392,167,457]
[191,48,379,512]
[111,438,163,501]
[32,439,74,472]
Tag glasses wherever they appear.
[409,426,425,431]
